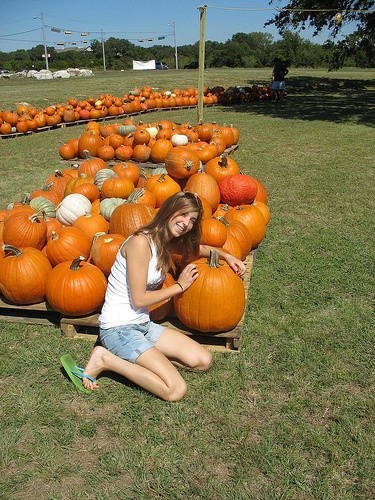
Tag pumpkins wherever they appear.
[60,119,239,162]
[203,84,272,104]
[0,147,270,333]
[0,86,198,134]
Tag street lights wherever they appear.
[33,11,49,70]
[166,21,179,69]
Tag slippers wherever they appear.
[60,354,97,395]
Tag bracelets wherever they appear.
[175,282,183,293]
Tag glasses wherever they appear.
[178,192,202,206]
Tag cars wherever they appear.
[155,61,169,70]
[183,61,199,70]
[0,66,10,74]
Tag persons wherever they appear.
[81,191,247,402]
[270,57,289,103]
